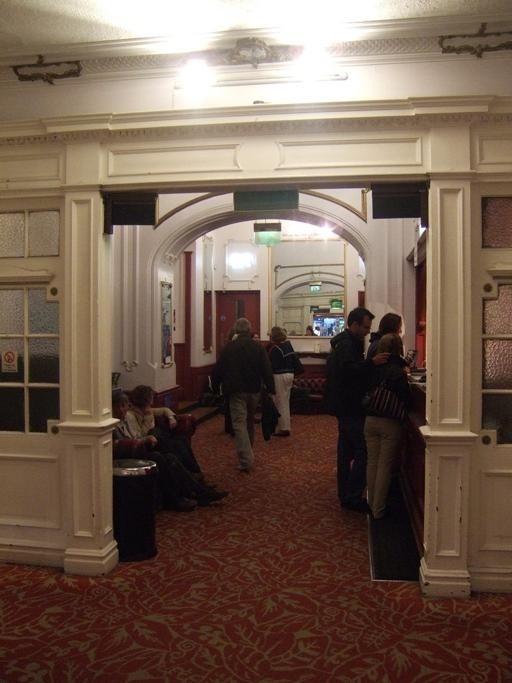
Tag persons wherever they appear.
[112,394,229,511]
[251,332,262,424]
[268,327,296,437]
[223,327,239,433]
[208,318,276,474]
[365,312,402,395]
[363,334,408,521]
[305,325,316,335]
[124,385,217,488]
[323,307,390,515]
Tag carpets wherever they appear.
[0,397,510,683]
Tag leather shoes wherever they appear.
[273,430,290,438]
[339,495,367,510]
[198,490,231,505]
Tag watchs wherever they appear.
[168,415,174,419]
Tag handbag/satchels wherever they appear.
[293,350,305,375]
[369,366,406,419]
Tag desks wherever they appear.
[396,386,425,555]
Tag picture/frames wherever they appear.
[160,280,173,369]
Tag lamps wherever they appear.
[329,299,344,313]
[309,266,322,293]
[254,219,281,245]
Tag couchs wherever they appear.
[113,390,197,513]
[293,365,329,391]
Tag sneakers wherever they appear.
[167,495,196,513]
[239,463,249,473]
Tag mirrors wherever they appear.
[268,233,348,339]
